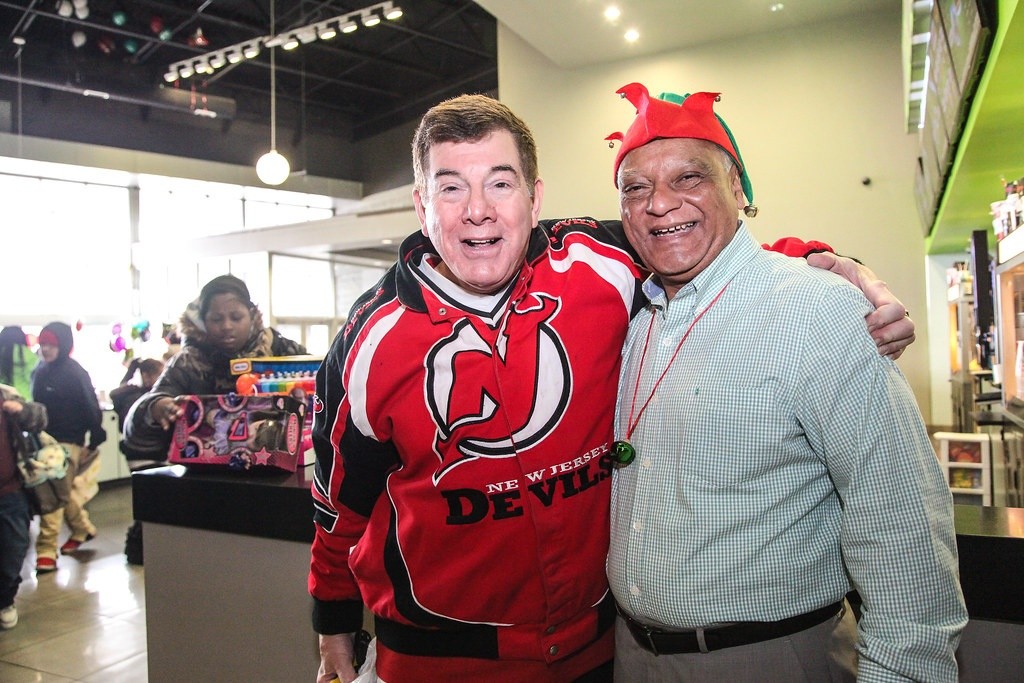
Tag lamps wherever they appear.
[253,1,290,186]
[163,0,403,84]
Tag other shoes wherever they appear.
[60,529,96,553]
[35,558,56,571]
[0,604,18,629]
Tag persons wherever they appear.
[110,359,165,566]
[124,273,310,461]
[0,384,48,628]
[309,98,915,683]
[0,326,37,401]
[604,81,974,683]
[32,322,106,570]
[163,324,182,361]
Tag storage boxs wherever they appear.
[229,353,324,466]
[167,393,307,474]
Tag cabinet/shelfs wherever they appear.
[933,431,992,507]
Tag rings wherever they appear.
[905,310,909,316]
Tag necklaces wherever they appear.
[611,287,727,463]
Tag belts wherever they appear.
[609,590,844,656]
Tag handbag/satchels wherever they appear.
[6,413,72,514]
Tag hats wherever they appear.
[37,330,59,346]
[605,83,756,218]
